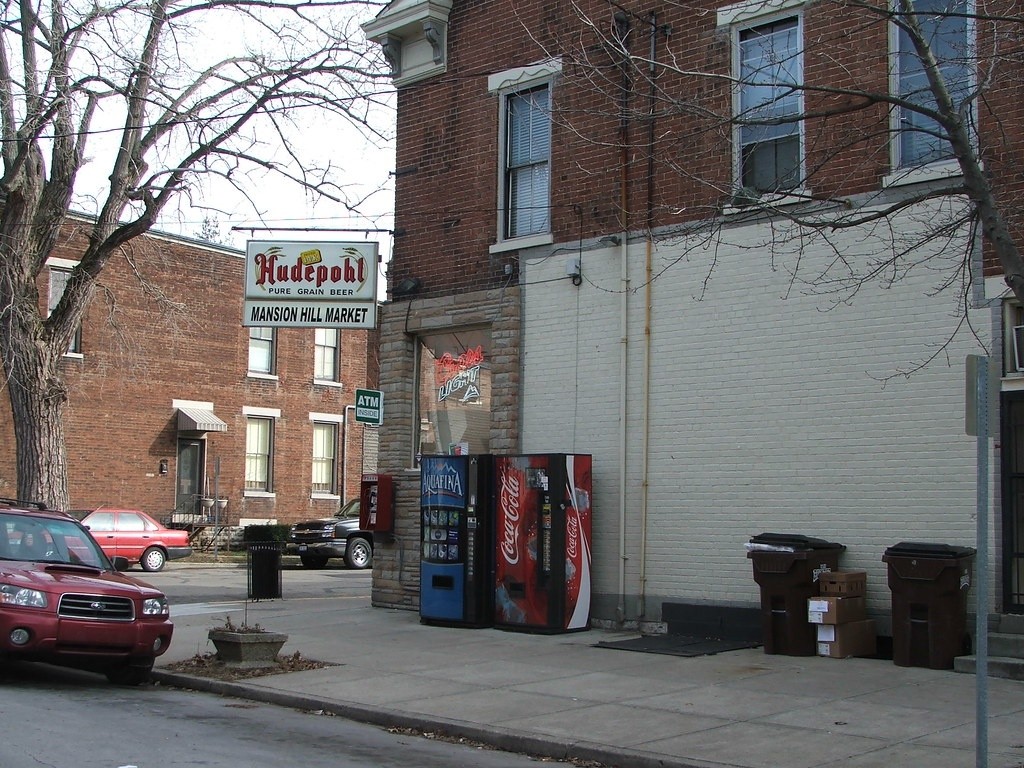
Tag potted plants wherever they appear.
[208,616,289,661]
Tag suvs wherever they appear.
[0,507,176,687]
[291,497,375,570]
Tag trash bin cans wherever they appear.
[746,532,847,657]
[240,539,286,600]
[882,541,977,669]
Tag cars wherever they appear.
[7,507,192,573]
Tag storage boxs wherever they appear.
[807,597,866,625]
[816,618,877,659]
[818,571,866,598]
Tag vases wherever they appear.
[201,498,215,507]
[218,500,228,508]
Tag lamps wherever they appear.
[399,278,420,292]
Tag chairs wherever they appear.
[19,533,48,559]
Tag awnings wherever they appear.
[178,408,228,432]
